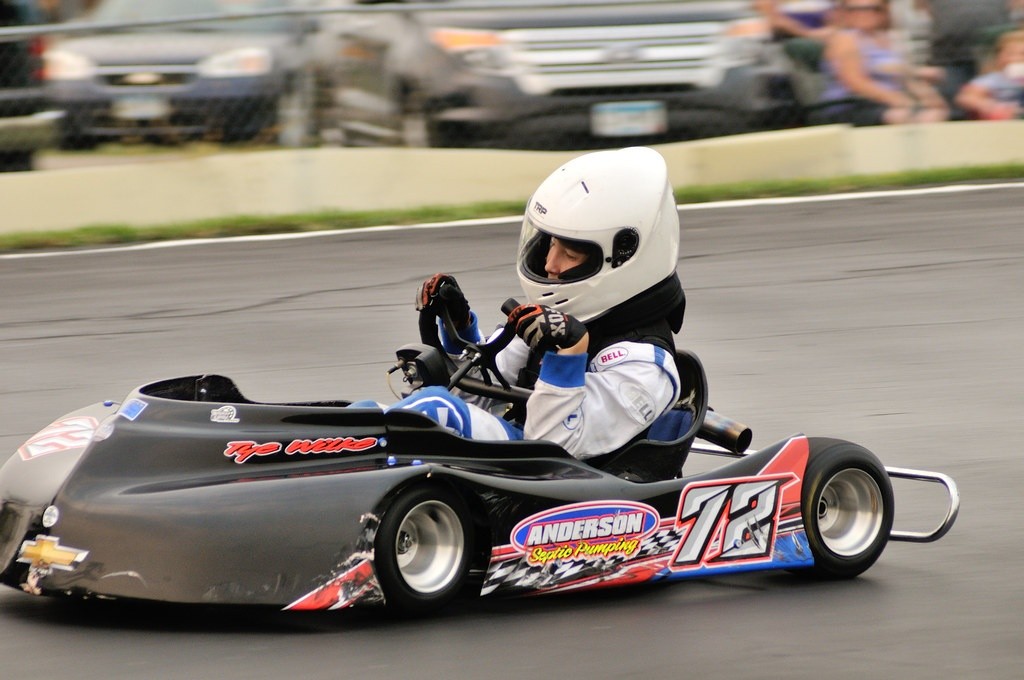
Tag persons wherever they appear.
[754,0,1024,127]
[342,145,685,463]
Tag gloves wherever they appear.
[412,274,470,325]
[506,303,588,355]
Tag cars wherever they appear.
[37,1,325,149]
[389,0,770,146]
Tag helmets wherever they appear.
[518,147,683,324]
